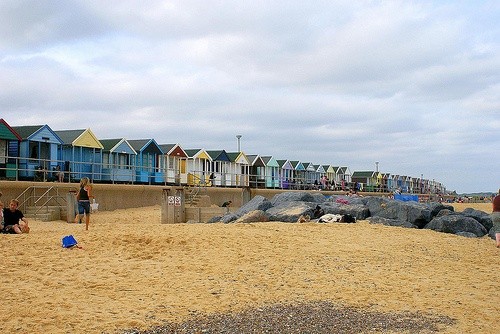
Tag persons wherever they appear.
[390,186,402,195]
[492,189,500,248]
[354,182,358,194]
[76,177,96,230]
[2,199,30,234]
[35,165,47,182]
[222,201,232,207]
[289,179,304,190]
[314,175,345,191]
[209,173,216,186]
[438,195,443,203]
[54,165,64,183]
[0,192,4,233]
[452,193,495,203]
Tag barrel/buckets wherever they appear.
[90,198,99,210]
[63,234,77,248]
